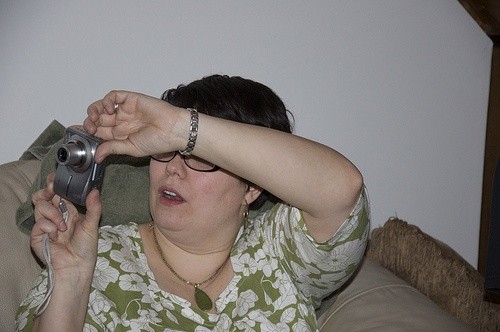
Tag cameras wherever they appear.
[52,126,106,207]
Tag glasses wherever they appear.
[149,151,220,172]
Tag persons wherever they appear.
[14,74,370,332]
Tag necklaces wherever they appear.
[152,219,231,312]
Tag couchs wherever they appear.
[0,121,500,332]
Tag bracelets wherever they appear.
[178,108,198,155]
